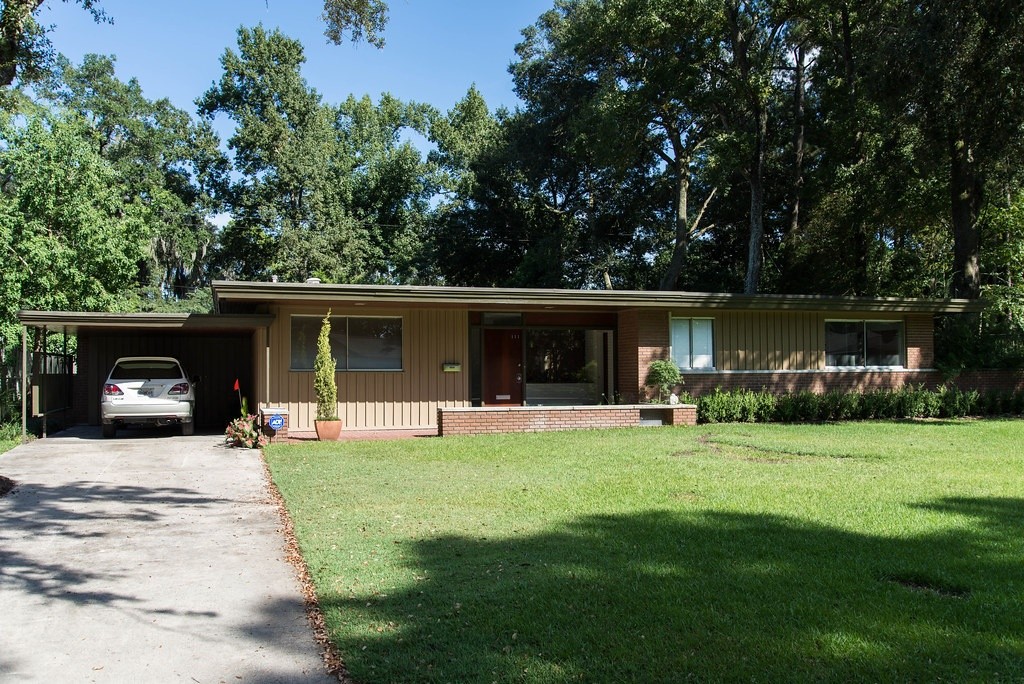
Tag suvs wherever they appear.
[98,356,197,435]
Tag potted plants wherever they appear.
[313,307,342,441]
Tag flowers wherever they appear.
[227,414,267,449]
